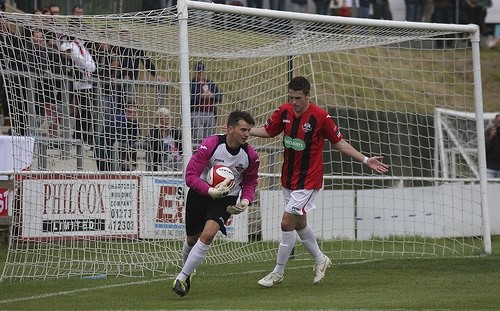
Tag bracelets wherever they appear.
[362,156,368,164]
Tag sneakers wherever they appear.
[181,270,197,295]
[258,267,288,289]
[310,255,335,285]
[170,271,185,296]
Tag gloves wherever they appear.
[206,177,236,200]
[225,201,256,216]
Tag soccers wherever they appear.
[207,164,234,189]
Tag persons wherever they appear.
[0,0,222,171]
[484,114,500,178]
[222,76,388,288]
[213,0,497,50]
[171,111,261,297]
